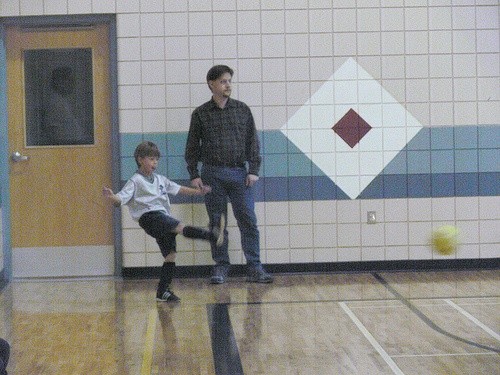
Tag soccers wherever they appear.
[432,225,459,254]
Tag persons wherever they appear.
[102,142,225,302]
[185,65,274,284]
[41,67,87,145]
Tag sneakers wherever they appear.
[156,289,182,303]
[211,213,225,247]
[211,266,230,283]
[246,266,273,283]
